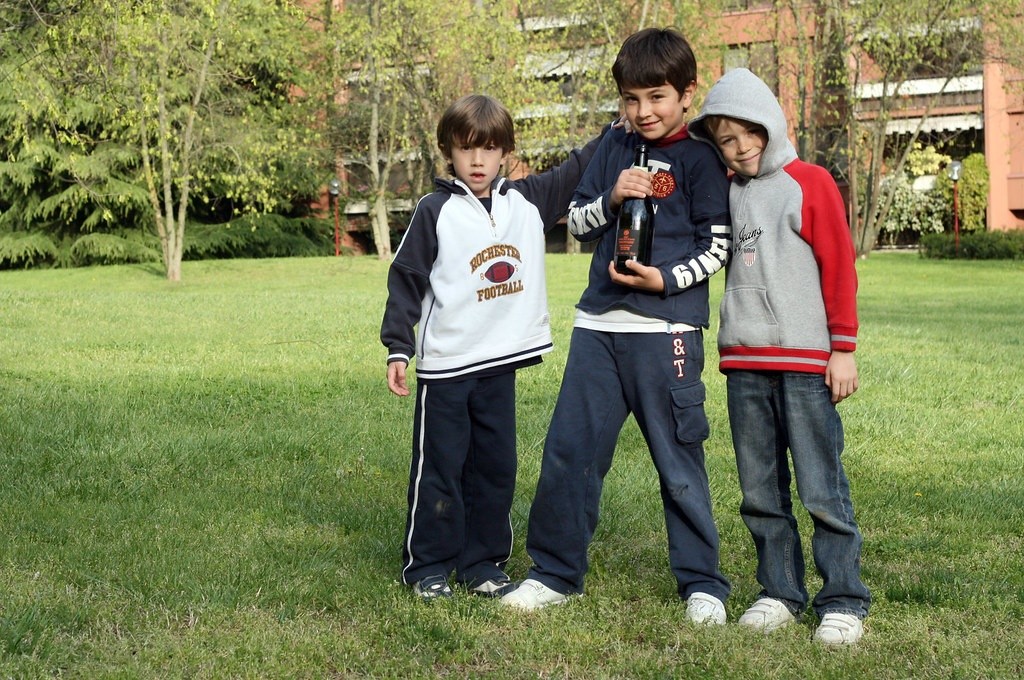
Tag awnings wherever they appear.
[851,73,984,98]
[504,12,596,31]
[852,15,982,42]
[514,45,620,79]
[343,198,414,214]
[514,96,621,119]
[340,62,430,84]
[343,147,423,164]
[885,112,984,135]
[513,135,595,155]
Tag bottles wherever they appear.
[614,145,654,276]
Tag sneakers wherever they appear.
[814,611,864,649]
[471,574,519,598]
[413,575,453,607]
[738,596,796,634]
[499,579,584,613]
[686,591,727,630]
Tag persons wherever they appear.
[686,67,871,647]
[380,94,628,606]
[498,28,732,628]
